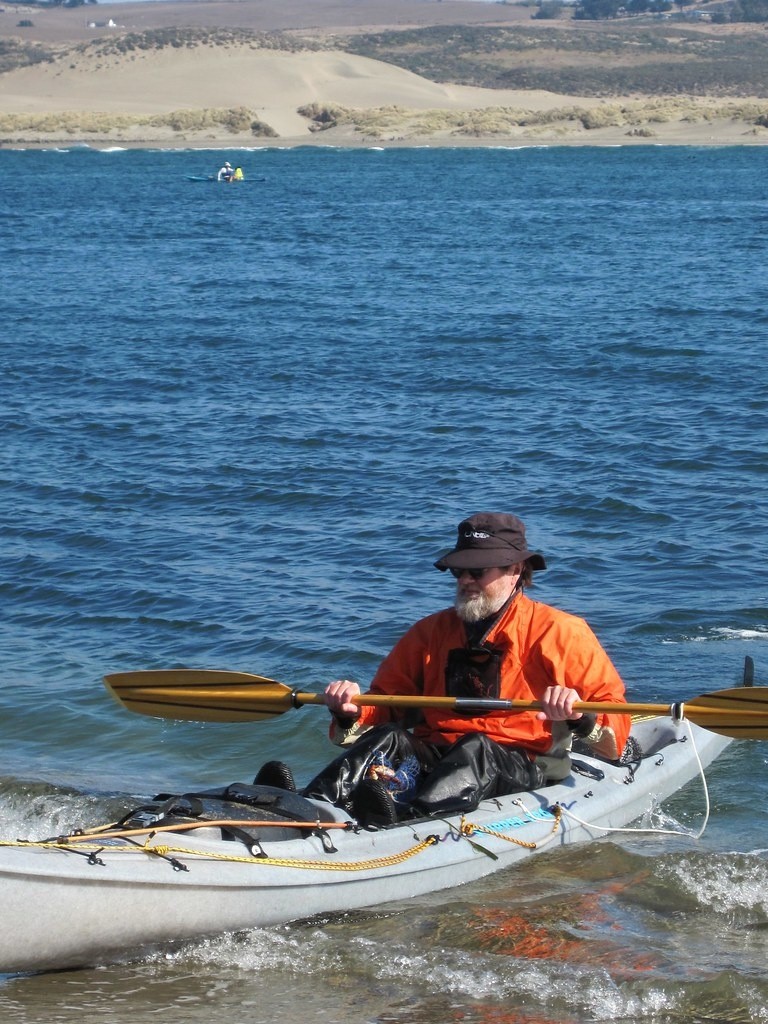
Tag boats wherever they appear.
[2,707,749,975]
[185,171,265,185]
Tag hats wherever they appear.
[431,511,545,572]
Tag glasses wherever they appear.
[449,567,488,578]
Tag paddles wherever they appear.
[102,669,768,741]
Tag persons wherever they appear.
[254,512,631,829]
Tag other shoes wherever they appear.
[253,761,297,793]
[352,780,397,829]
